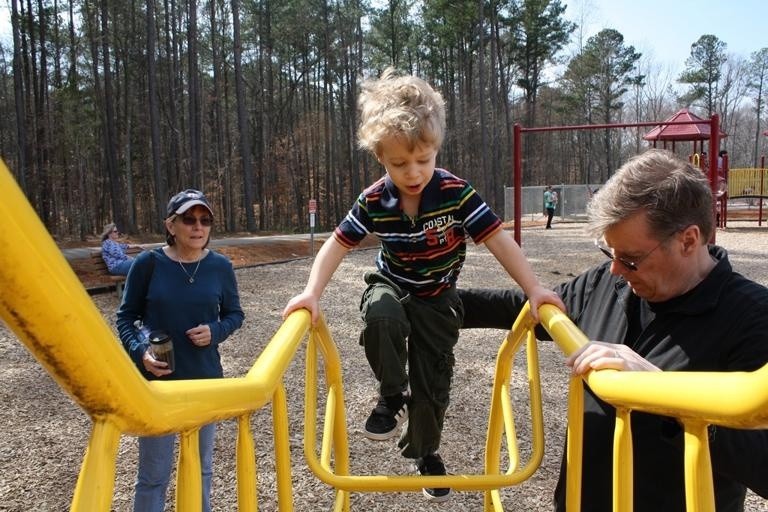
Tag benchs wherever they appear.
[90,246,145,298]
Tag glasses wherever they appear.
[113,230,118,233]
[181,216,212,226]
[596,229,678,273]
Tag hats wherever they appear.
[166,189,214,219]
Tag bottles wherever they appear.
[129,316,151,350]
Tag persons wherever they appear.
[456,146,768,512]
[544,186,558,228]
[717,151,727,169]
[743,186,756,205]
[283,69,567,502]
[116,191,245,512]
[101,223,145,275]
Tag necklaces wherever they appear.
[178,258,200,283]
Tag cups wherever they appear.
[147,327,175,376]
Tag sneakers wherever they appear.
[411,452,450,503]
[364,382,411,441]
